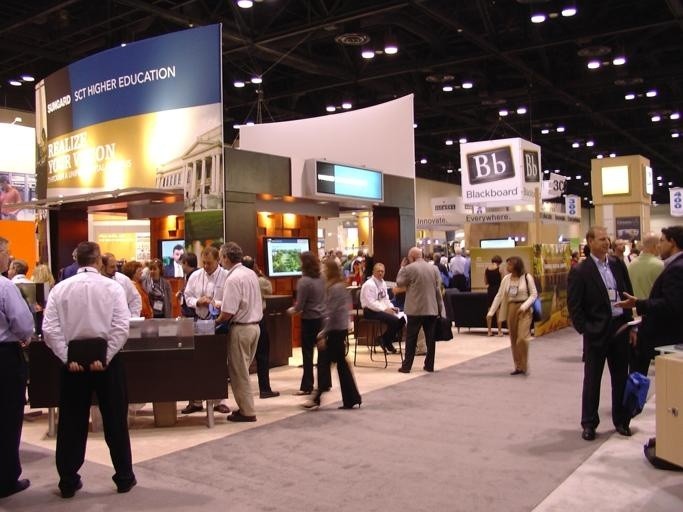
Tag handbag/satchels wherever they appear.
[533,296,543,322]
[436,287,446,319]
[435,317,453,341]
[622,371,650,418]
[441,272,450,288]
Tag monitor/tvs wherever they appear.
[157,239,185,279]
[263,236,311,278]
[480,238,516,247]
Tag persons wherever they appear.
[2,247,171,320]
[484,256,504,336]
[1,237,32,499]
[396,246,442,373]
[569,225,683,440]
[360,263,404,352]
[41,242,136,498]
[487,256,538,375]
[287,246,369,410]
[424,242,471,292]
[177,241,279,423]
[163,244,187,278]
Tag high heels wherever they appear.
[338,398,362,409]
[299,401,320,409]
[291,386,313,395]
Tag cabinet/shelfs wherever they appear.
[262,297,293,368]
[654,344,683,467]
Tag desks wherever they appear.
[28,334,228,436]
[444,289,502,333]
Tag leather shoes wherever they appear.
[616,421,631,436]
[377,335,397,353]
[117,478,137,493]
[582,426,597,440]
[61,478,82,498]
[0,478,29,498]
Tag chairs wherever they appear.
[353,293,404,368]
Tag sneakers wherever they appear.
[180,404,203,413]
[424,366,433,371]
[260,390,279,398]
[511,369,522,375]
[398,367,410,373]
[226,407,257,422]
[213,404,230,413]
